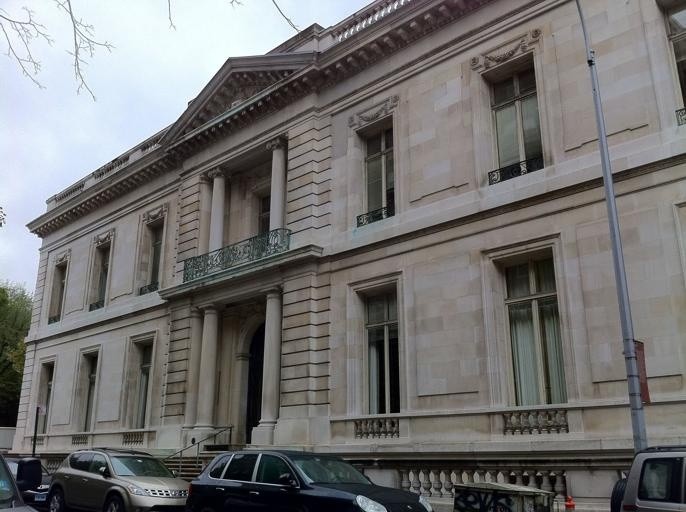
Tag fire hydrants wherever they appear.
[563,495,575,511]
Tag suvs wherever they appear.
[46,447,190,511]
[185,450,435,512]
[608,445,686,511]
[0,452,43,512]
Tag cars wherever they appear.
[3,457,52,505]
